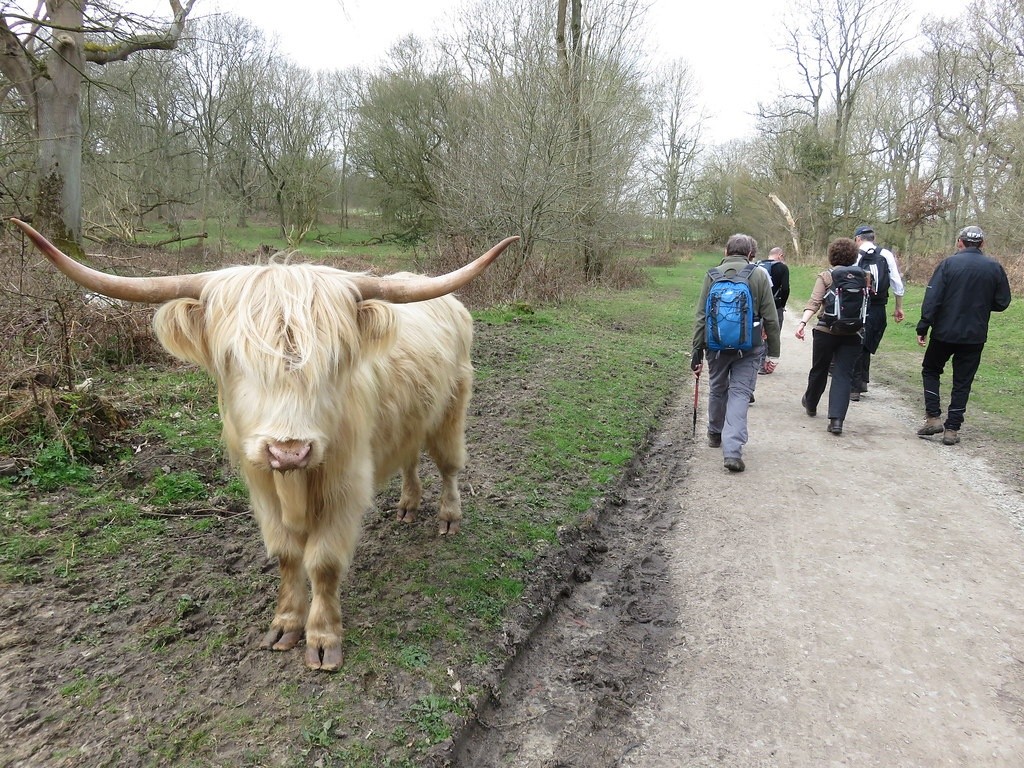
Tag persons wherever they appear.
[691,233,781,472]
[795,238,876,435]
[849,225,904,402]
[754,247,790,375]
[915,225,1012,444]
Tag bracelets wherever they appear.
[800,321,807,326]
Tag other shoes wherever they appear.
[707,430,721,447]
[724,457,745,471]
[749,394,755,402]
[758,369,765,374]
[850,392,860,401]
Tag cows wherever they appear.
[12,218,523,675]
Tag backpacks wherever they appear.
[706,264,758,359]
[757,260,783,277]
[857,247,890,304]
[822,266,869,334]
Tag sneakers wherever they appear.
[827,418,843,433]
[917,415,944,435]
[943,428,960,444]
[802,394,817,416]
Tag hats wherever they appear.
[854,226,874,241]
[958,226,985,242]
